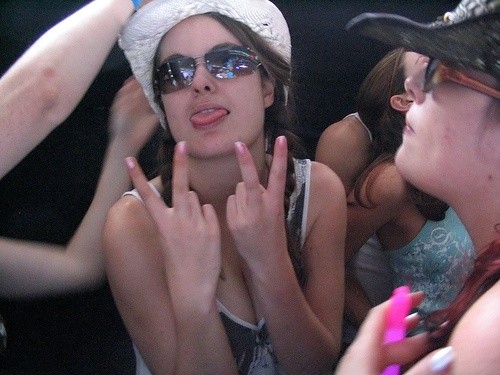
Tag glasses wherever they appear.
[153,44,268,94]
[422,58,500,98]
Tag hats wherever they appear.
[119,0,292,129]
[346,1,500,78]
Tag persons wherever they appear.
[343,46,480,337]
[333,0,500,374]
[98,1,347,375]
[0,0,160,301]
[314,113,394,358]
